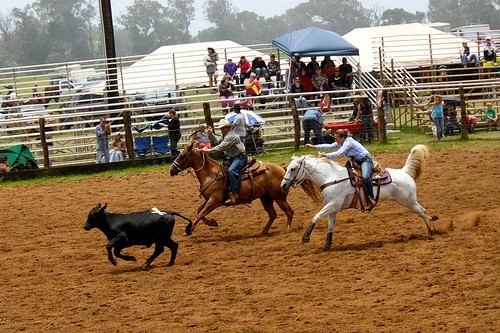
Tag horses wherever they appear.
[280,145,439,252]
[169,140,324,236]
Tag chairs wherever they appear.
[132,136,153,165]
[151,135,171,163]
[241,123,265,153]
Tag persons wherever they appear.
[232,103,246,143]
[287,55,353,116]
[0,155,39,180]
[32,121,53,156]
[459,42,478,68]
[96,116,124,164]
[302,108,325,145]
[484,103,500,131]
[483,37,498,66]
[203,47,281,114]
[159,110,182,155]
[32,84,38,97]
[431,96,477,141]
[352,97,374,145]
[305,129,375,200]
[6,85,13,94]
[202,119,248,203]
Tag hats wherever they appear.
[485,36,491,39]
[214,119,234,128]
[232,104,241,109]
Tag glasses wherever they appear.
[220,127,224,129]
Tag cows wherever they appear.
[84,203,192,267]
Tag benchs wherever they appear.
[0,44,401,167]
[373,31,500,135]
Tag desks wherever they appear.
[323,119,386,140]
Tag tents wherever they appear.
[90,39,290,127]
[272,27,360,93]
[280,23,484,74]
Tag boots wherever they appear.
[224,194,239,203]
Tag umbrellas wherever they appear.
[226,109,265,127]
[441,96,461,107]
[0,144,34,170]
